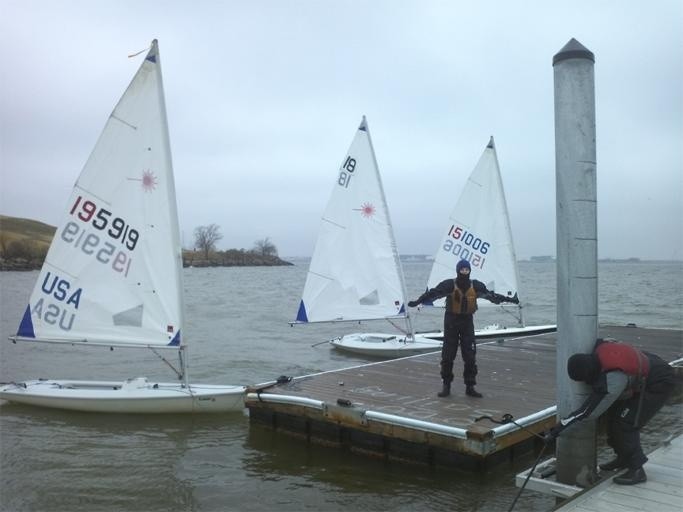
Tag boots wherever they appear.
[438,378,449,397]
[465,383,483,397]
[599,450,632,471]
[613,463,647,485]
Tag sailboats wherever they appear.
[1,40,248,413]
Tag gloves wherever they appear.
[408,297,426,307]
[504,296,519,304]
[542,427,557,445]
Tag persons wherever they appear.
[547,339,677,485]
[408,258,520,398]
